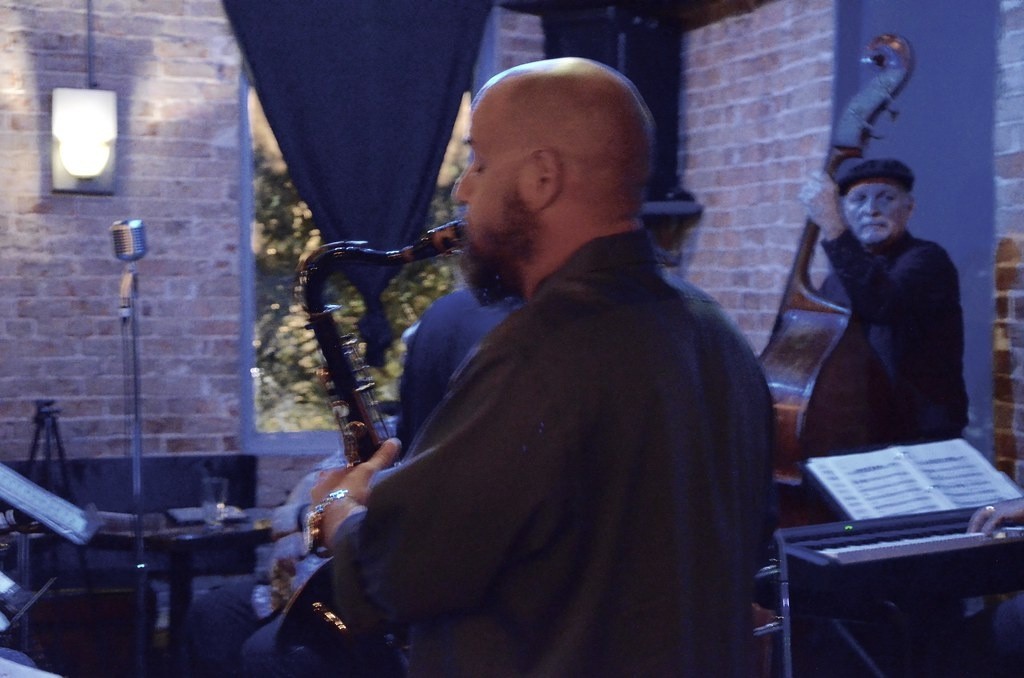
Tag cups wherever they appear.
[200,477,230,530]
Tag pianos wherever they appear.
[774,502,1024,591]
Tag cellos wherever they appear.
[755,32,915,678]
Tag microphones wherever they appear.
[108,217,148,264]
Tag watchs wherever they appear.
[303,488,349,554]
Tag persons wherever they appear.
[796,155,970,455]
[966,497,1024,657]
[180,54,778,678]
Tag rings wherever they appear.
[986,505,995,512]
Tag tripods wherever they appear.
[13,410,78,533]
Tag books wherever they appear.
[806,439,1022,522]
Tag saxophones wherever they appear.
[273,219,465,678]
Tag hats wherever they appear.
[837,158,914,190]
